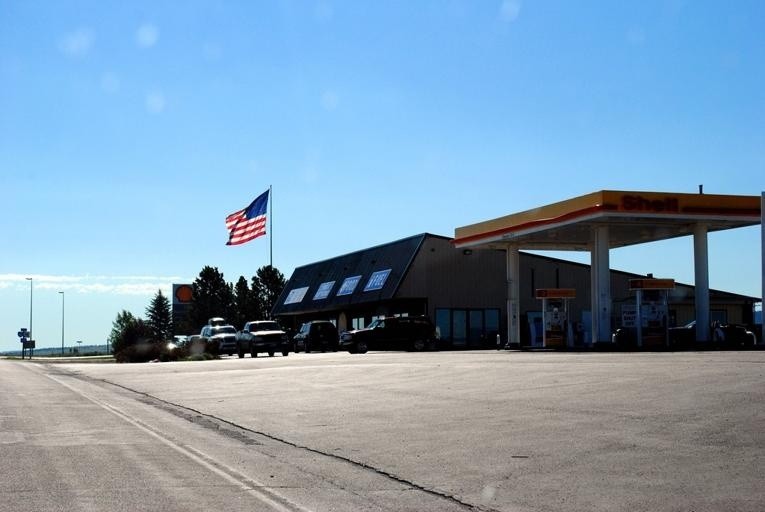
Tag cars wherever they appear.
[609,325,637,342]
[668,320,756,346]
[174,335,202,346]
[292,321,339,352]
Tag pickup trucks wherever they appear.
[234,320,289,357]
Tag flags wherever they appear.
[225,189,268,246]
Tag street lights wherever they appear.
[59,291,64,352]
[26,278,32,341]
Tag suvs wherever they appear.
[341,316,434,353]
[201,318,238,356]
